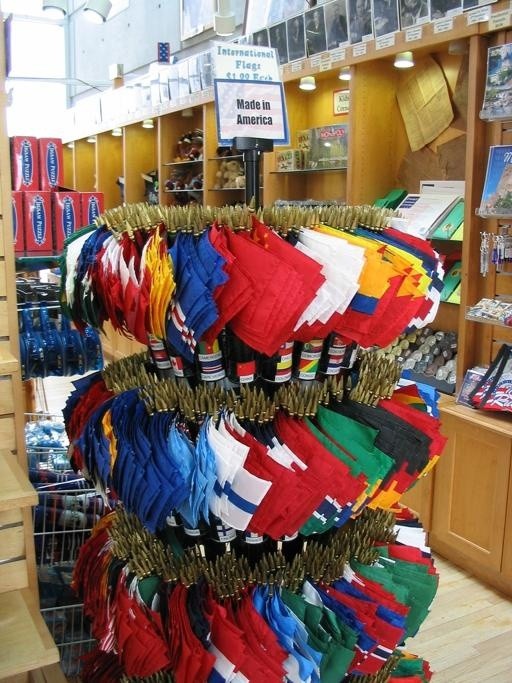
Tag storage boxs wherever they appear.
[10,135,105,257]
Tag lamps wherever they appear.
[298,77,316,91]
[214,0,236,36]
[338,66,351,80]
[83,0,112,23]
[42,0,67,19]
[182,108,193,117]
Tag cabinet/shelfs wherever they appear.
[160,106,203,212]
[65,118,160,211]
[428,408,510,578]
[461,26,511,415]
[350,35,473,406]
[202,101,266,212]
[0,10,65,683]
[100,319,148,360]
[266,66,352,212]
